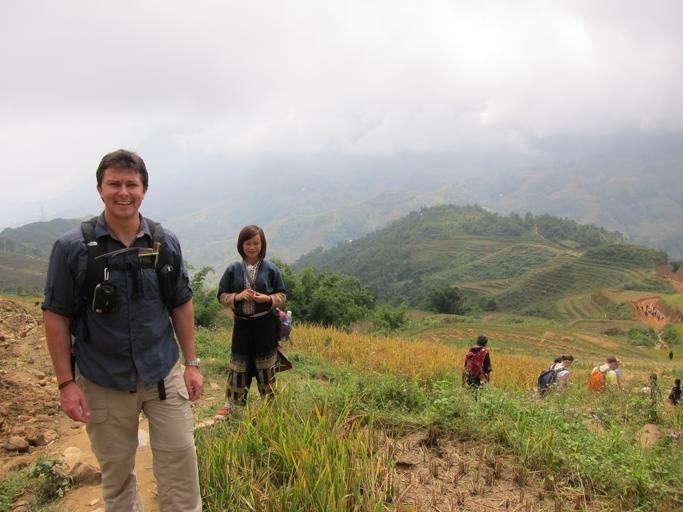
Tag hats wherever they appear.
[606,356,622,363]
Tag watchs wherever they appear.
[184,359,199,366]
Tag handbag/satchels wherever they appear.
[240,259,292,341]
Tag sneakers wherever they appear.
[214,407,235,421]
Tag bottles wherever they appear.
[280,311,292,341]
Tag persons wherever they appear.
[649,374,664,424]
[669,379,682,406]
[669,350,673,359]
[590,357,618,394]
[538,356,573,399]
[42,149,205,511]
[462,336,492,388]
[216,225,285,419]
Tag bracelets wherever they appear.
[57,379,75,390]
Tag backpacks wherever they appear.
[590,366,614,392]
[539,362,568,398]
